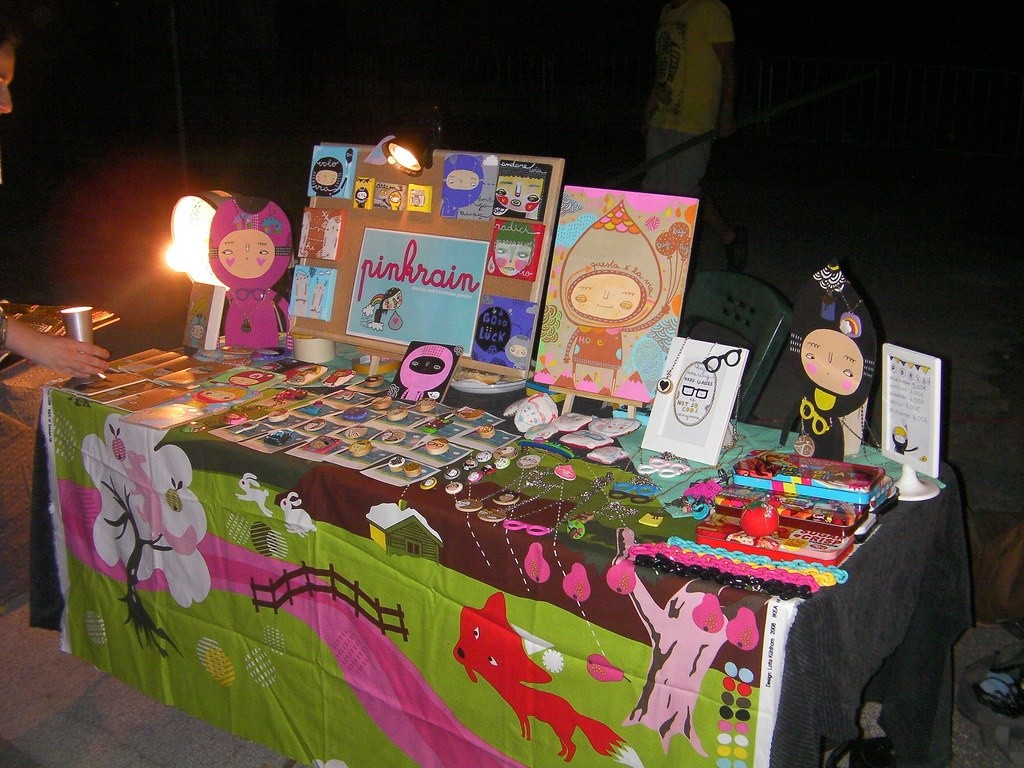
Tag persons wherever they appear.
[0,31,109,379]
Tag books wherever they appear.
[54,280,287,431]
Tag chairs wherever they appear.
[678,270,793,422]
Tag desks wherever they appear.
[35,341,976,768]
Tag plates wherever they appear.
[450,378,526,394]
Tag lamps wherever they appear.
[61,306,96,386]
[172,189,237,348]
[384,105,444,178]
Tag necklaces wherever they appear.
[234,289,270,331]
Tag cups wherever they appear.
[59,307,97,385]
